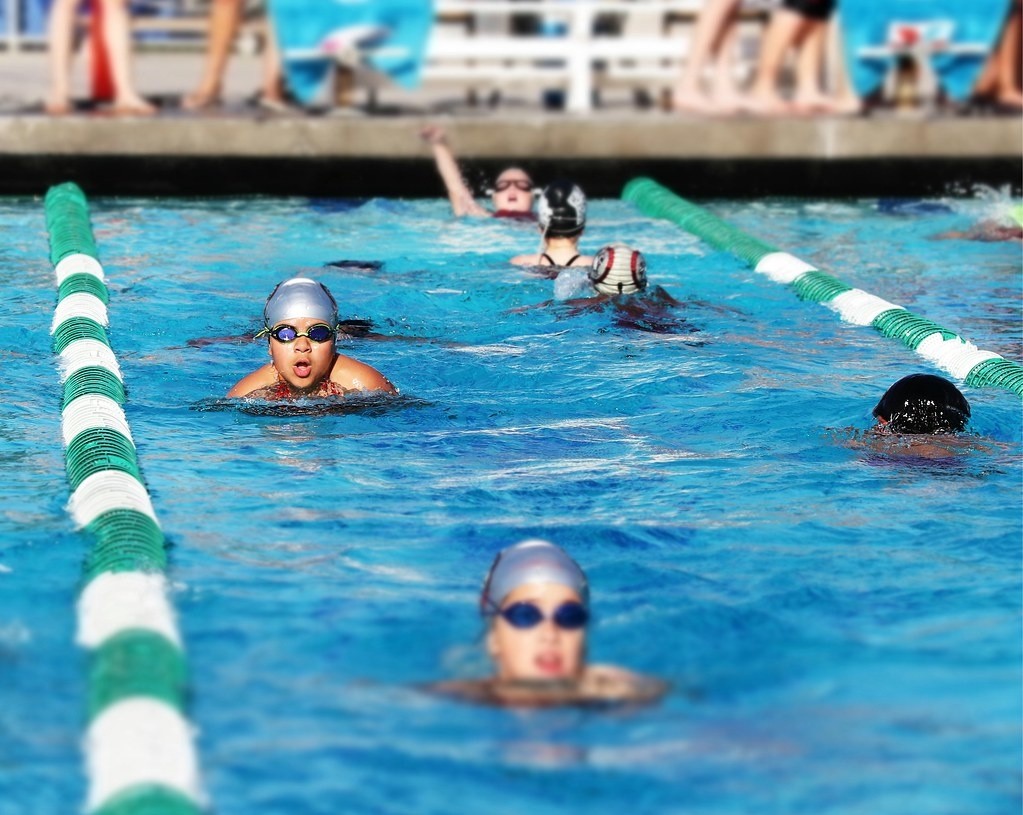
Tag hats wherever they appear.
[538,182,587,234]
[482,539,590,614]
[872,373,971,434]
[264,278,339,330]
[590,244,647,295]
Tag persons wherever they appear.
[480,538,637,683]
[227,277,398,400]
[872,373,970,434]
[177,0,294,115]
[38,0,159,119]
[552,242,647,300]
[970,0,1023,110]
[416,124,542,217]
[668,0,749,115]
[745,0,857,119]
[507,182,596,266]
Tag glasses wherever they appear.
[482,602,588,629]
[494,180,532,191]
[255,323,340,343]
